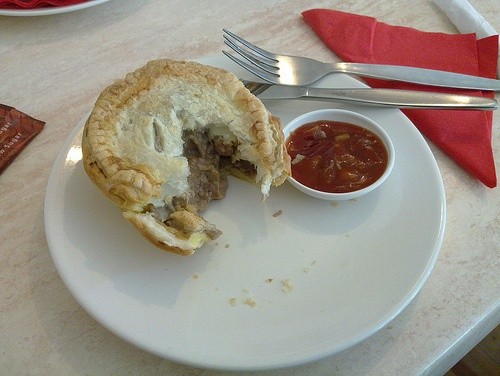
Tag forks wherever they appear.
[219,24,499,93]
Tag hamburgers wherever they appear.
[81,58,293,255]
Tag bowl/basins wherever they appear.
[277,108,396,200]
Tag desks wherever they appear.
[0,1,500,376]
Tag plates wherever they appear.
[44,51,449,371]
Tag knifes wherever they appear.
[229,76,499,114]
